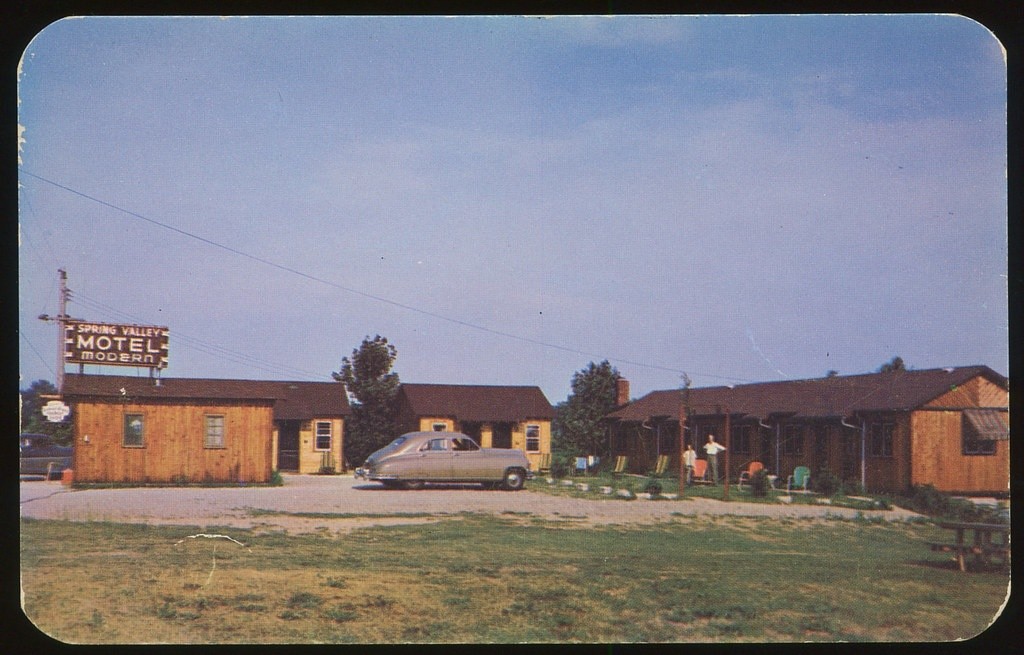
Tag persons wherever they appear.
[702,434,725,486]
[684,445,697,487]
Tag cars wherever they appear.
[355,430,529,490]
[20,434,73,480]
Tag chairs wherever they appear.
[537,453,553,475]
[656,454,670,475]
[691,460,710,481]
[738,462,763,491]
[787,465,811,494]
[610,455,630,480]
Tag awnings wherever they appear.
[963,409,1008,441]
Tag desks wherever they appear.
[940,520,1010,574]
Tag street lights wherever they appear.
[38,313,66,395]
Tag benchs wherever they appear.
[922,539,1011,572]
